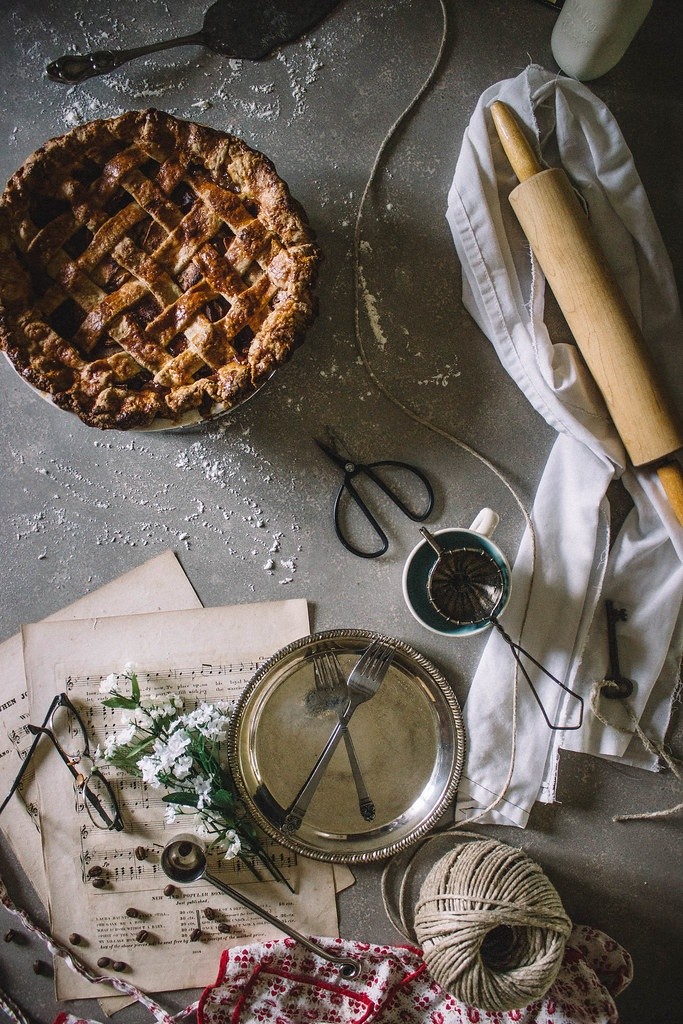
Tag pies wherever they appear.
[0,109,324,428]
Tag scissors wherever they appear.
[311,424,434,558]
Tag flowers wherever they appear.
[92,660,297,895]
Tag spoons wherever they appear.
[468,507,500,539]
[47,0,341,84]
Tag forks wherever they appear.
[280,637,397,836]
[312,652,376,823]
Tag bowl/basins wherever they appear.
[402,527,514,638]
[228,630,465,863]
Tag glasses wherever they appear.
[1,694,125,833]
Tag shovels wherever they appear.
[46,0,346,85]
